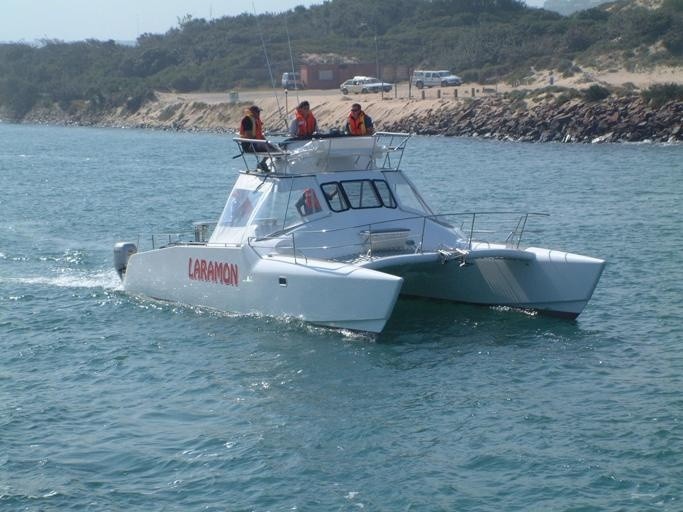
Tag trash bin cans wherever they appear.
[196,224,209,242]
[229,92,238,101]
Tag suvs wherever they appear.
[413,70,461,89]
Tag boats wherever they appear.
[111,11,608,335]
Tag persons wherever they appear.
[342,104,375,137]
[239,105,289,153]
[288,100,321,139]
[294,186,338,218]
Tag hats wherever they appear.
[250,106,263,113]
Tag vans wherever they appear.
[281,72,302,90]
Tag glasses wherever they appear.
[351,109,359,112]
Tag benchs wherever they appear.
[232,136,288,176]
[360,228,414,260]
[373,127,412,169]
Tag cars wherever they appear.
[339,76,392,94]
